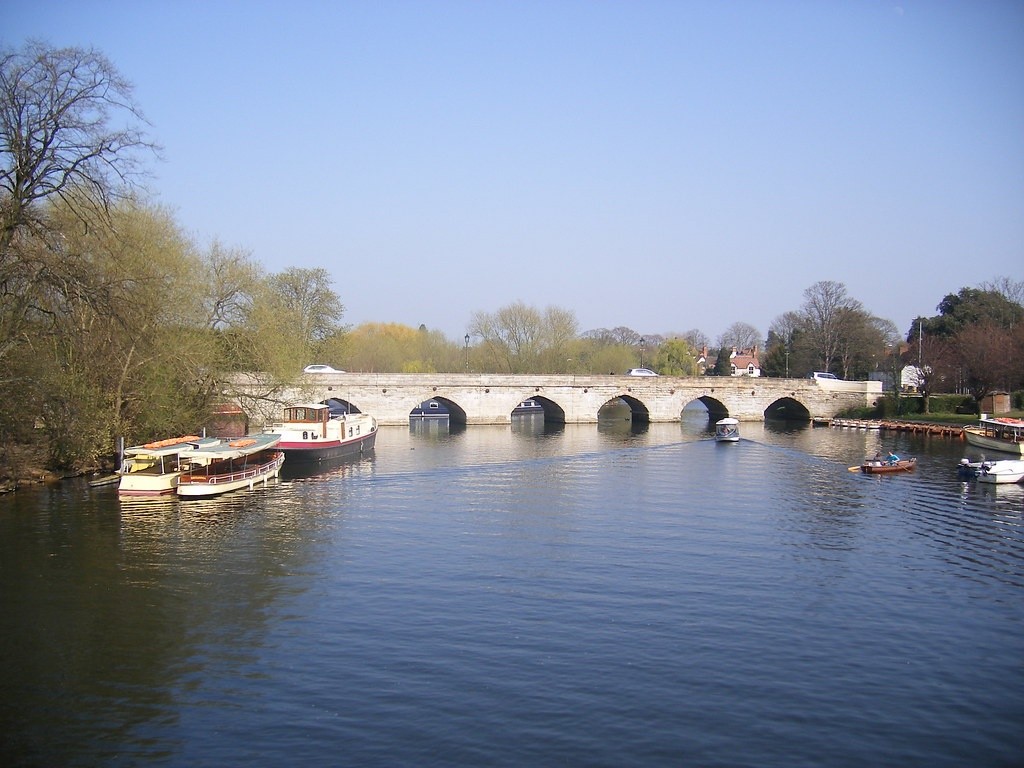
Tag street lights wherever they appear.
[464,334,470,373]
[639,338,646,369]
[784,348,790,379]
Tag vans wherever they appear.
[806,371,842,381]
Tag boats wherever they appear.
[959,414,1024,456]
[118,432,285,500]
[957,458,1024,484]
[861,457,917,473]
[714,418,740,442]
[261,395,379,464]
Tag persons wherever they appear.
[874,452,884,465]
[886,452,900,467]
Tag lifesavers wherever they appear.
[144,436,198,448]
[228,439,255,448]
[994,417,1020,423]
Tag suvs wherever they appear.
[303,364,347,374]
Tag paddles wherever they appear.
[848,466,860,471]
[896,462,912,473]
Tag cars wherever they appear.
[624,368,659,377]
